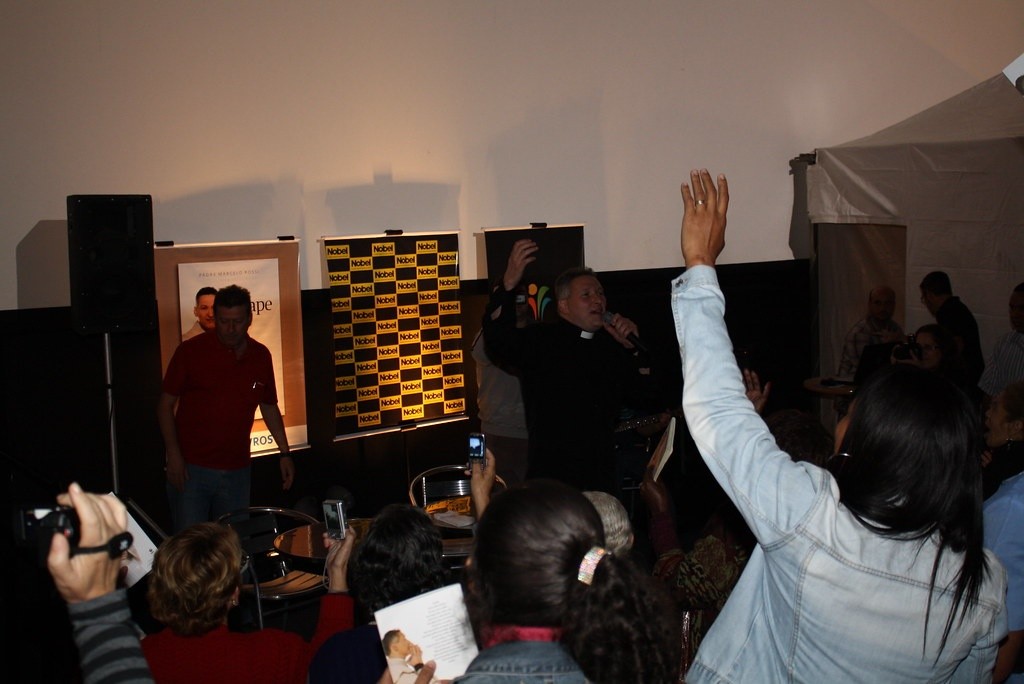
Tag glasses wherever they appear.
[921,344,941,353]
[921,294,932,306]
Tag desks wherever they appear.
[804,375,861,436]
[272,520,482,568]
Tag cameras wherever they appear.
[894,333,923,360]
[42,508,81,558]
[323,499,348,540]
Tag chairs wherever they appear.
[407,464,509,573]
[216,507,332,628]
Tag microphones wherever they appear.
[602,311,648,354]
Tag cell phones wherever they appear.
[469,433,486,474]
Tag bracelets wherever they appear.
[279,452,291,457]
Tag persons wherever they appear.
[838,284,906,376]
[890,281,1024,684]
[381,629,436,684]
[470,286,528,494]
[666,169,1009,684]
[918,269,986,368]
[180,287,220,341]
[157,285,291,535]
[0,370,834,684]
[481,239,640,497]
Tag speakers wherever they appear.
[67,194,157,334]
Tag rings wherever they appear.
[695,200,705,204]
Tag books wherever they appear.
[374,582,480,684]
[106,491,159,587]
[647,416,676,482]
